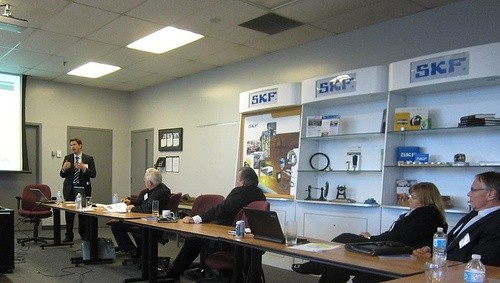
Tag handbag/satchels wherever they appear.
[346,241,412,256]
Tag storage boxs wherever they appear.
[82,237,116,262]
[306,116,322,138]
[320,114,342,136]
[396,186,412,207]
[398,145,420,165]
[414,153,429,164]
[393,107,429,131]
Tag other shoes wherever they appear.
[292,262,322,275]
[131,248,139,265]
[62,238,73,242]
[157,268,180,280]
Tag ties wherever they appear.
[448,210,479,245]
[73,157,80,185]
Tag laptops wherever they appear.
[243,207,308,243]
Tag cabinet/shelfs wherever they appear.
[233,41,500,280]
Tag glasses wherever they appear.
[470,187,496,193]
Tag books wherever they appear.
[458,113,500,125]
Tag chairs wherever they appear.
[15,184,57,247]
[185,194,225,281]
[122,192,182,265]
[195,200,270,283]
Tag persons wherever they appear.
[291,182,448,283]
[60,138,96,243]
[155,167,266,278]
[110,168,175,272]
[411,171,500,267]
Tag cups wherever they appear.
[285,221,298,245]
[425,260,448,283]
[162,210,175,219]
[152,200,159,216]
[86,197,93,208]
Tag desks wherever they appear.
[36,201,500,283]
[379,262,500,283]
[0,206,15,273]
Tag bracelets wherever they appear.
[62,167,64,171]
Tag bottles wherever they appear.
[463,254,486,283]
[432,227,447,265]
[57,191,62,206]
[112,194,118,204]
[75,193,82,210]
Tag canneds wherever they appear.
[235,220,245,238]
